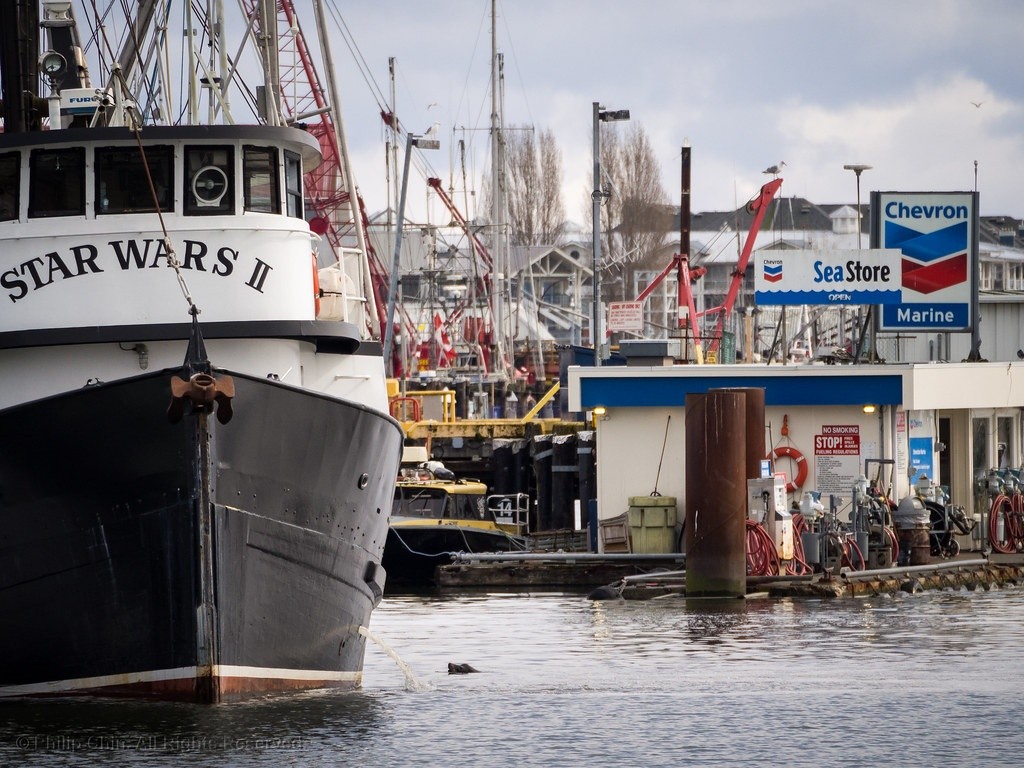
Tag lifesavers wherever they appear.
[312,253,321,316]
[766,447,810,493]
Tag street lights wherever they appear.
[379,133,443,383]
[843,164,872,360]
[591,100,631,364]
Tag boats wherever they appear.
[1,0,408,717]
[388,442,531,586]
